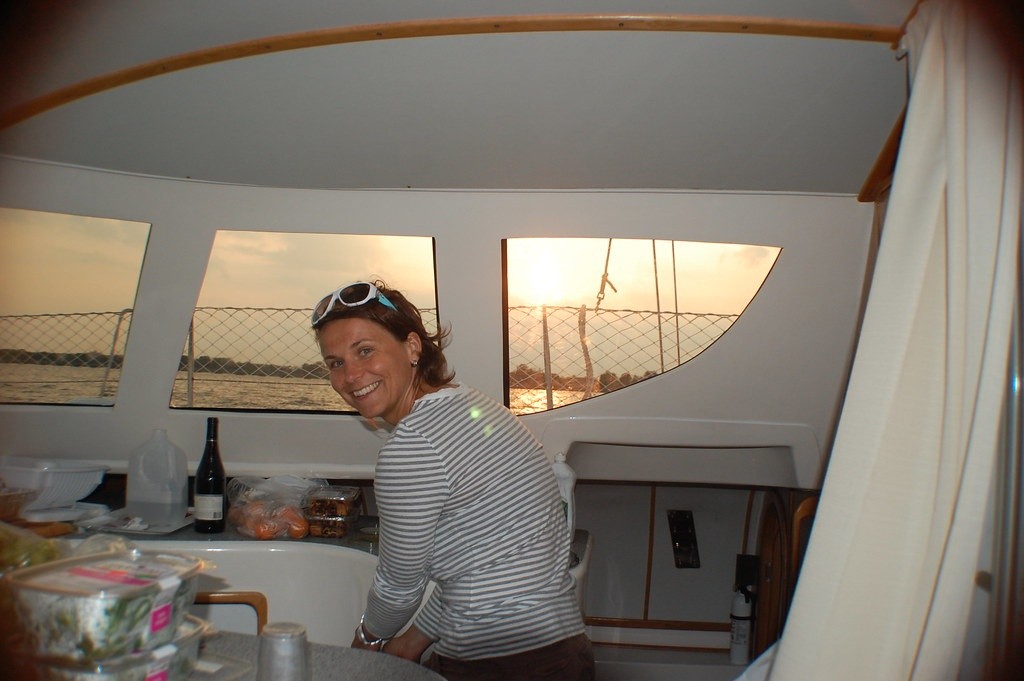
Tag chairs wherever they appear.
[194,592,267,634]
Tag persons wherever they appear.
[309,282,597,681]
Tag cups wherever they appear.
[256,621,308,681]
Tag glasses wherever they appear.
[310,281,399,325]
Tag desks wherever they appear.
[188,631,448,681]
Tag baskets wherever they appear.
[0,457,111,511]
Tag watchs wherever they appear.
[355,611,383,646]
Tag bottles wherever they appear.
[126,428,188,526]
[193,417,226,532]
[729,586,752,665]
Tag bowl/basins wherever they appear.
[3,546,216,681]
[304,484,360,539]
